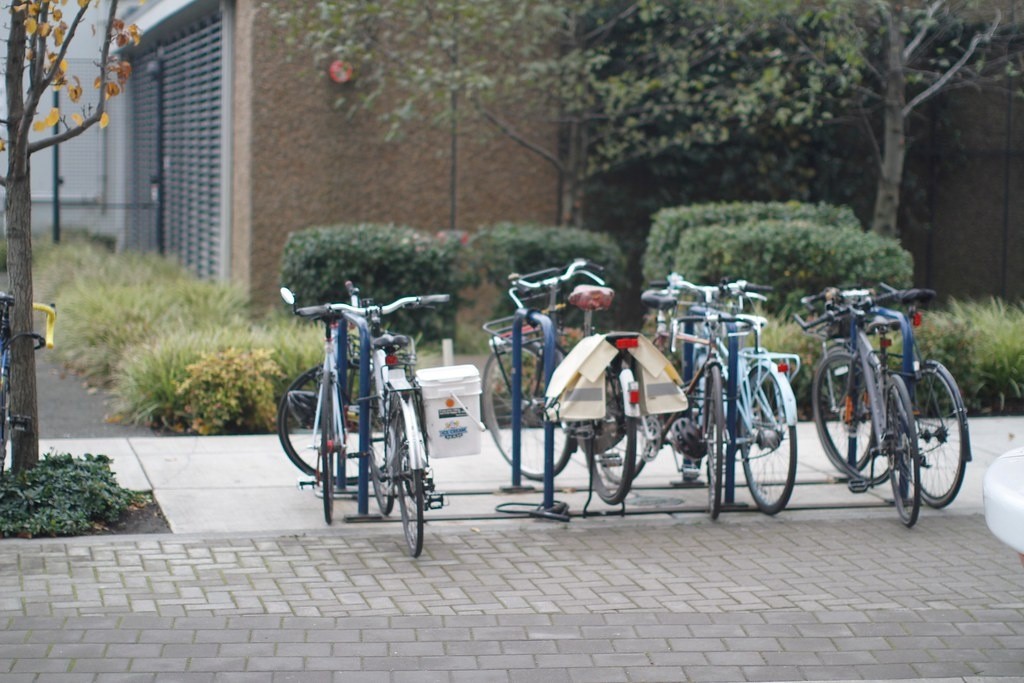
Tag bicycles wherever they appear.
[642,273,800,519]
[801,288,971,510]
[278,280,383,527]
[0,291,58,477]
[481,258,640,520]
[280,286,452,558]
[810,289,938,526]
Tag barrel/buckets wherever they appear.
[415,364,486,459]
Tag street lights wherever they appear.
[146,56,166,254]
[52,82,60,243]
